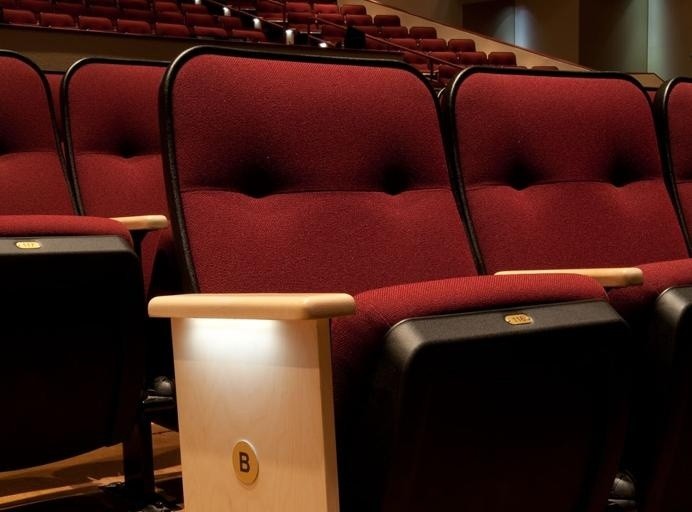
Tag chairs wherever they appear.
[1,2,692,511]
